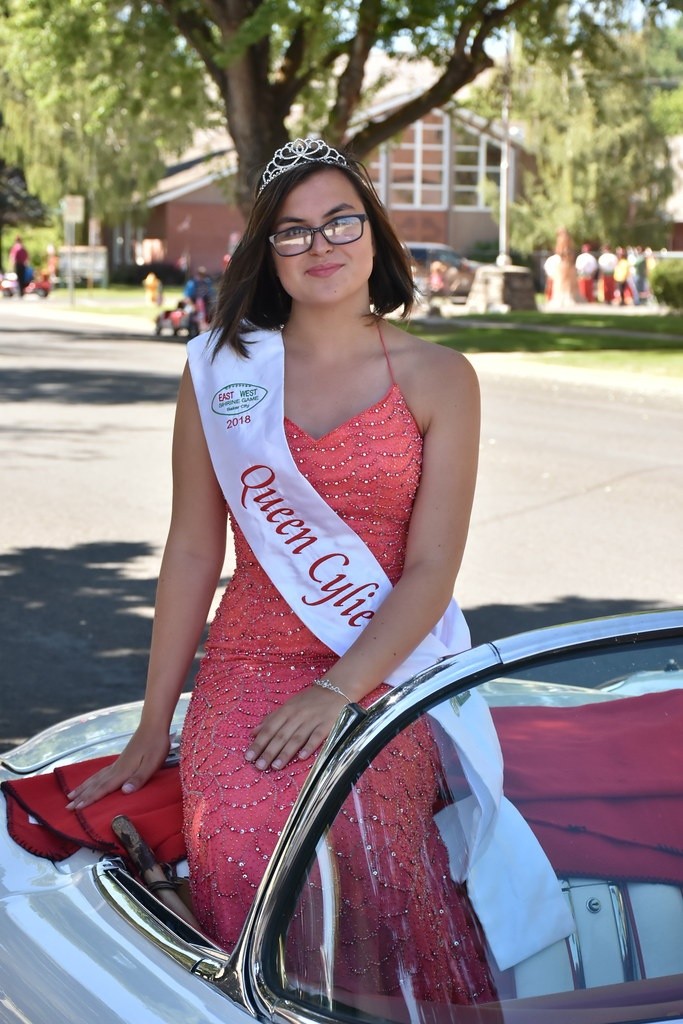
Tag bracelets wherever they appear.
[311,678,353,705]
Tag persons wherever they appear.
[418,241,677,308]
[7,236,27,295]
[176,266,213,337]
[61,139,501,1022]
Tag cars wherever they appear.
[399,241,486,299]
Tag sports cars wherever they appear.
[0,607,683,1024]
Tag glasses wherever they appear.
[268,213,370,258]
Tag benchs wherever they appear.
[156,311,200,341]
[0,688,683,996]
